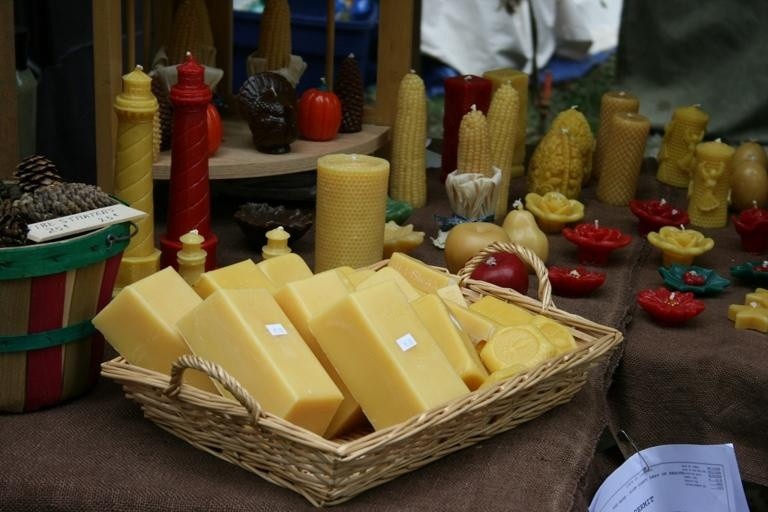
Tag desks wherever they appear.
[0,138,768,512]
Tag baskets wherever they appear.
[98,242,626,507]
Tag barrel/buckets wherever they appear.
[0,178,138,418]
